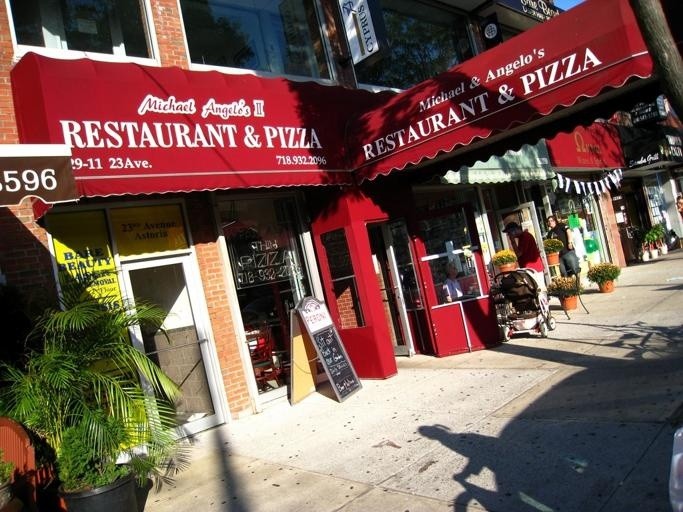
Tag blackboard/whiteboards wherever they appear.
[311,324,363,403]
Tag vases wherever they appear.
[599,279,614,293]
[559,295,578,310]
[498,263,517,274]
[546,251,560,265]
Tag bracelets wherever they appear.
[567,241,573,245]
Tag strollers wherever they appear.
[491,264,555,342]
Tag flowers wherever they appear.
[490,249,518,266]
[586,262,620,288]
[546,277,584,299]
[543,239,564,254]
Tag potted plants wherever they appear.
[1,251,199,512]
[640,221,669,262]
[0,450,25,512]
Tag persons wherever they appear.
[547,216,579,278]
[442,262,463,302]
[677,196,683,219]
[502,222,545,290]
